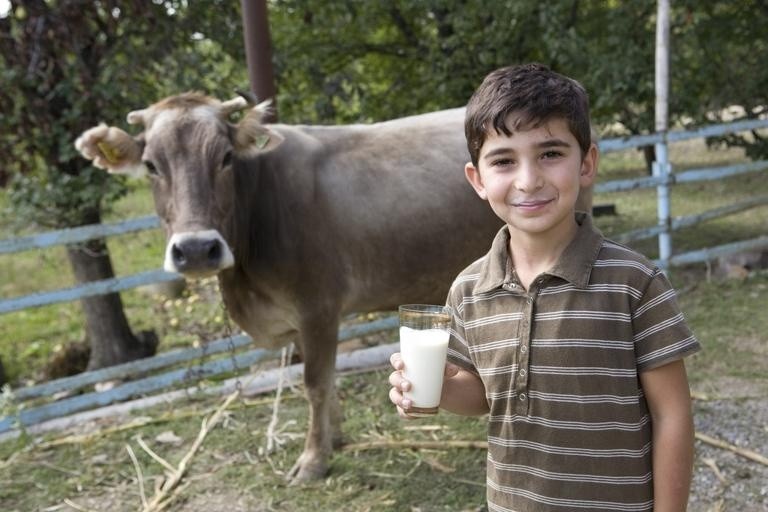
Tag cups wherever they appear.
[397,303,453,420]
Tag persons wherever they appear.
[385,61,693,510]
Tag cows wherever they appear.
[72,88,600,488]
